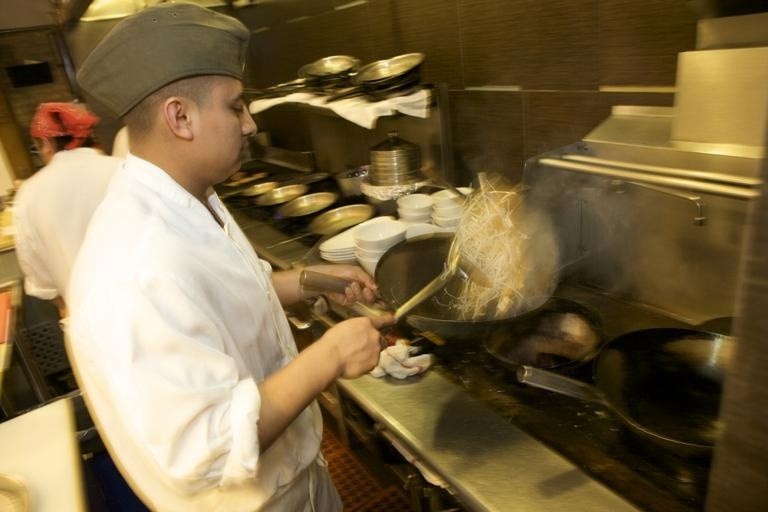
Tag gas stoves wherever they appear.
[415,289,724,512]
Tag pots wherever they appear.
[298,230,561,342]
[217,181,376,250]
[516,327,734,454]
[238,52,426,108]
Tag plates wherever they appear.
[318,215,396,265]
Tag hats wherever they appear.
[77,3,254,117]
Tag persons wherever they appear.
[59,0,390,511]
[7,101,114,369]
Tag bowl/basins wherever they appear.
[351,186,476,278]
